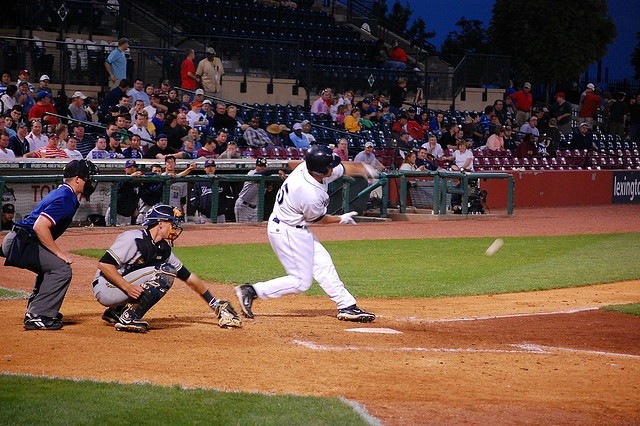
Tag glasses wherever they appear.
[228,142,235,144]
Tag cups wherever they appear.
[226,151,231,160]
[40,147,46,158]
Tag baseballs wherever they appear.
[487,238,504,256]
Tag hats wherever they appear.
[190,100,204,107]
[151,93,160,99]
[206,47,215,54]
[2,204,16,213]
[181,135,196,142]
[18,68,30,76]
[408,107,417,113]
[156,108,165,114]
[55,123,68,136]
[204,160,216,167]
[16,123,25,129]
[554,93,565,98]
[400,130,409,137]
[364,97,370,104]
[49,133,60,140]
[195,89,204,96]
[126,160,136,168]
[280,125,290,131]
[118,37,129,45]
[364,142,373,148]
[302,120,310,126]
[580,122,589,127]
[618,92,626,97]
[293,123,303,131]
[19,80,28,85]
[587,83,595,88]
[72,91,88,100]
[112,132,121,141]
[163,79,170,87]
[524,82,531,89]
[35,91,52,99]
[158,134,168,138]
[530,116,538,120]
[202,99,212,105]
[40,74,51,81]
[267,124,282,134]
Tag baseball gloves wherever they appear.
[216,301,242,328]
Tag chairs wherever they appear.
[181,0,411,80]
[65,38,131,66]
[373,149,403,170]
[209,127,245,142]
[593,132,640,165]
[193,124,208,140]
[240,102,391,148]
[469,147,600,171]
[435,149,454,170]
[243,146,310,160]
[430,110,486,126]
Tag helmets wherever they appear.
[306,145,341,175]
[141,202,183,247]
[62,160,99,202]
[256,158,266,167]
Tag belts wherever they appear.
[242,200,258,208]
[272,217,306,229]
[92,278,99,287]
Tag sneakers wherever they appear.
[336,304,375,323]
[102,306,123,322]
[234,284,258,319]
[114,317,150,333]
[24,312,63,331]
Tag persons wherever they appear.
[160,156,196,209]
[190,159,232,217]
[1,159,98,331]
[577,83,640,148]
[180,49,199,91]
[195,47,224,95]
[104,36,130,90]
[383,40,409,72]
[92,205,244,333]
[507,82,533,125]
[232,145,378,322]
[388,77,406,118]
[318,79,577,160]
[440,141,474,174]
[416,148,434,171]
[532,90,573,136]
[0,203,15,230]
[105,159,143,226]
[398,152,425,171]
[1,68,319,159]
[331,136,349,161]
[353,141,376,172]
[368,38,391,67]
[234,157,285,221]
[135,165,162,225]
